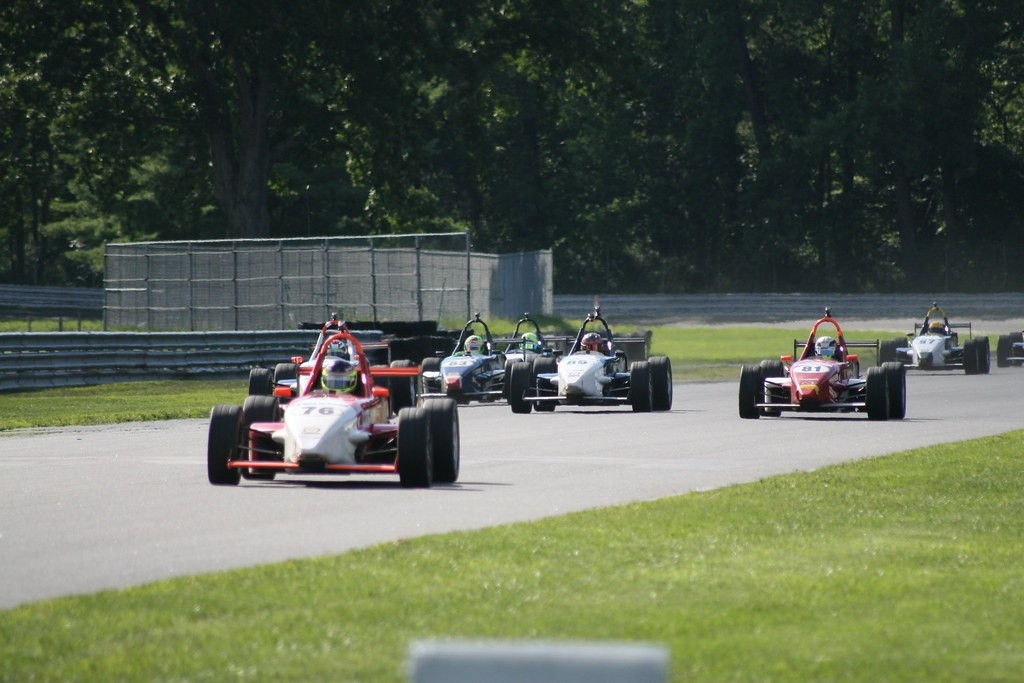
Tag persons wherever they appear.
[814,336,840,360]
[929,322,946,335]
[580,332,604,354]
[518,332,538,352]
[463,334,487,355]
[324,339,349,360]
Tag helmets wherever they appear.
[321,358,358,395]
[815,336,837,358]
[578,331,603,351]
[518,333,538,351]
[325,339,351,361]
[926,320,946,335]
[462,335,485,355]
